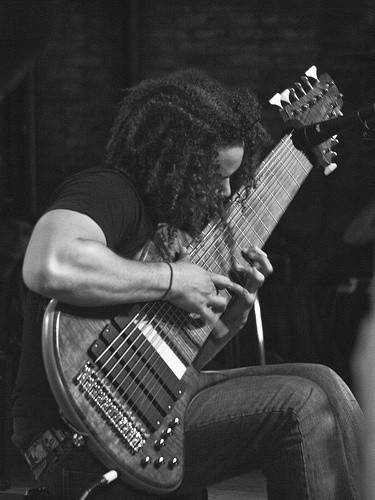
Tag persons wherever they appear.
[11,70,374,500]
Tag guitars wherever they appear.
[38,62,349,491]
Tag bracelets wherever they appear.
[159,261,174,301]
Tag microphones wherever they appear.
[292,103,375,151]
